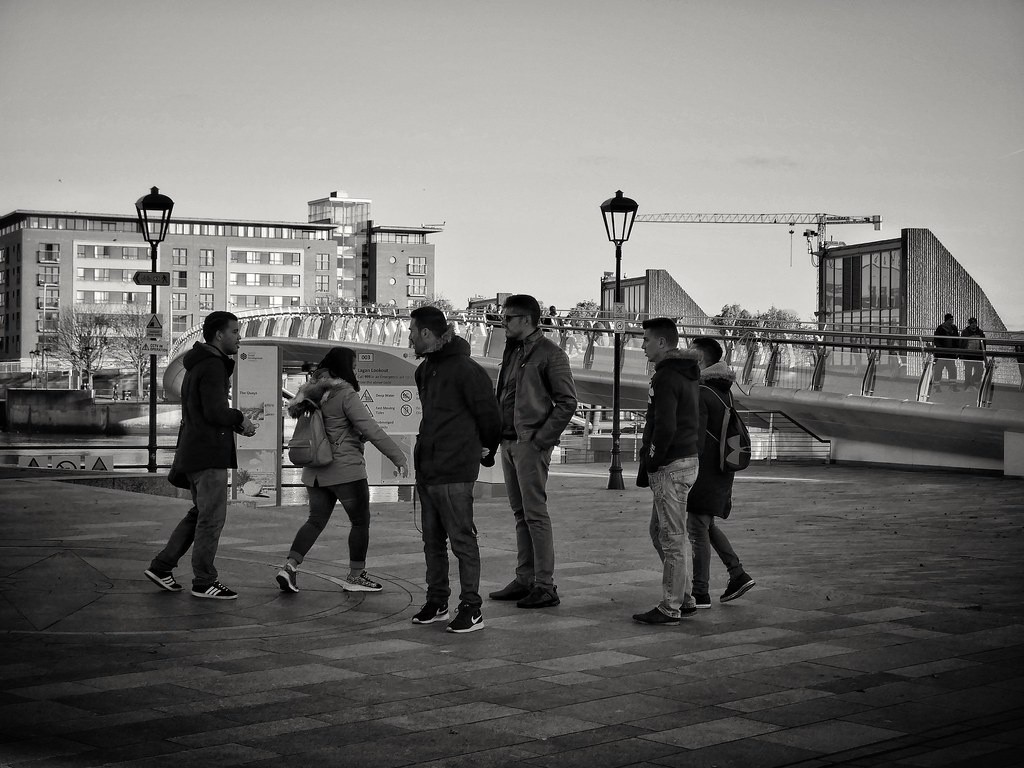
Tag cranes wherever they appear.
[632,212,884,333]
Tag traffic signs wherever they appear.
[133,272,171,286]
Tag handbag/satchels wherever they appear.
[167,424,191,489]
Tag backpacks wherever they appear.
[288,399,350,467]
[699,385,751,472]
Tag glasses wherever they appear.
[504,314,528,322]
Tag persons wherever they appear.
[630,318,701,624]
[485,295,578,608]
[959,317,986,392]
[932,314,960,392]
[542,305,564,332]
[112,383,120,401]
[487,303,505,328]
[408,307,504,633]
[275,346,410,592]
[562,316,583,358]
[81,382,90,390]
[685,338,754,609]
[143,311,257,599]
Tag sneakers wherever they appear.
[720,572,755,603]
[691,593,711,608]
[488,580,532,600]
[276,565,299,593]
[632,607,681,625]
[412,602,449,623]
[681,608,697,616]
[445,608,484,632]
[343,570,382,591]
[192,578,238,599]
[517,589,560,608]
[143,567,183,591]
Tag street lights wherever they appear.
[85,347,93,400]
[134,186,176,473]
[30,347,52,391]
[599,190,640,491]
